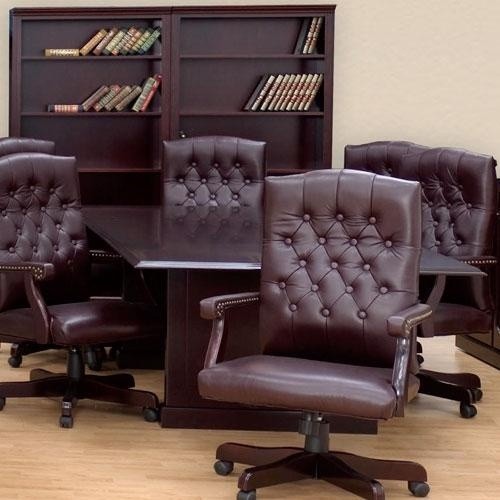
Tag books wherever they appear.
[292,17,311,55]
[47,72,163,111]
[243,74,323,111]
[302,17,318,54]
[308,17,323,54]
[44,25,162,55]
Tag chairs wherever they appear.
[2,137,61,157]
[343,141,423,178]
[0,154,159,429]
[399,147,499,416]
[164,135,268,208]
[198,167,430,498]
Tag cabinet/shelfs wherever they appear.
[9,7,168,368]
[165,3,336,184]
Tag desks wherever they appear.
[78,206,489,433]
